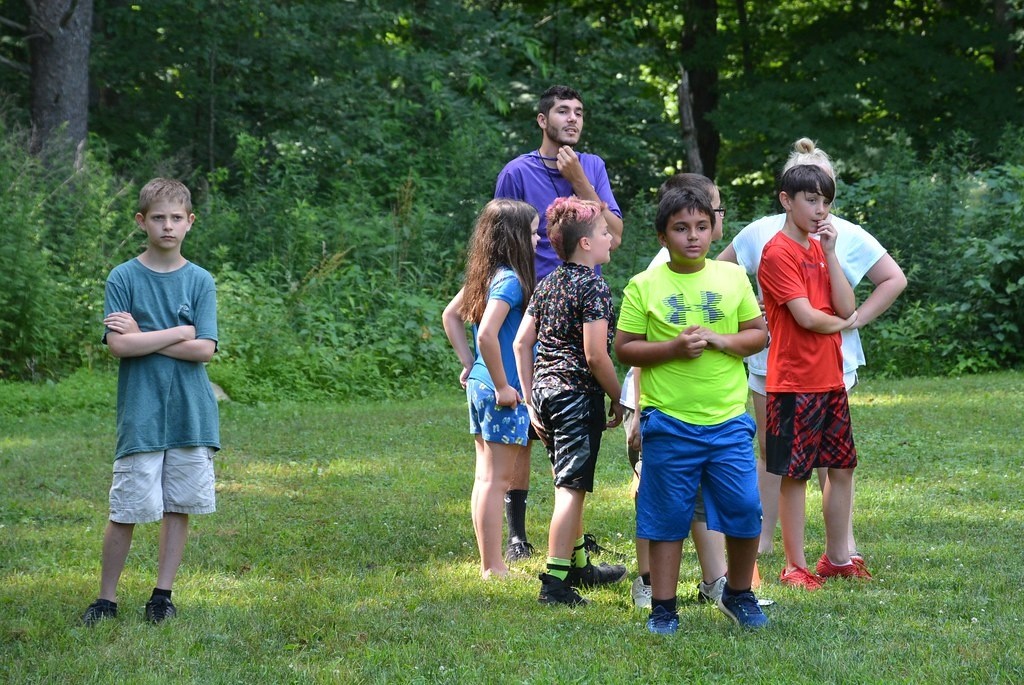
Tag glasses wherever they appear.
[713,208,726,219]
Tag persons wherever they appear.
[614,186,768,632]
[83,177,221,627]
[442,137,909,610]
[492,84,627,566]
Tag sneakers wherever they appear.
[718,588,768,632]
[646,605,680,634]
[780,563,825,592]
[146,596,176,626]
[538,573,590,609]
[83,603,117,629]
[815,553,872,580]
[630,576,652,609]
[505,541,535,563]
[697,575,727,605]
[570,556,628,593]
[584,533,626,564]
